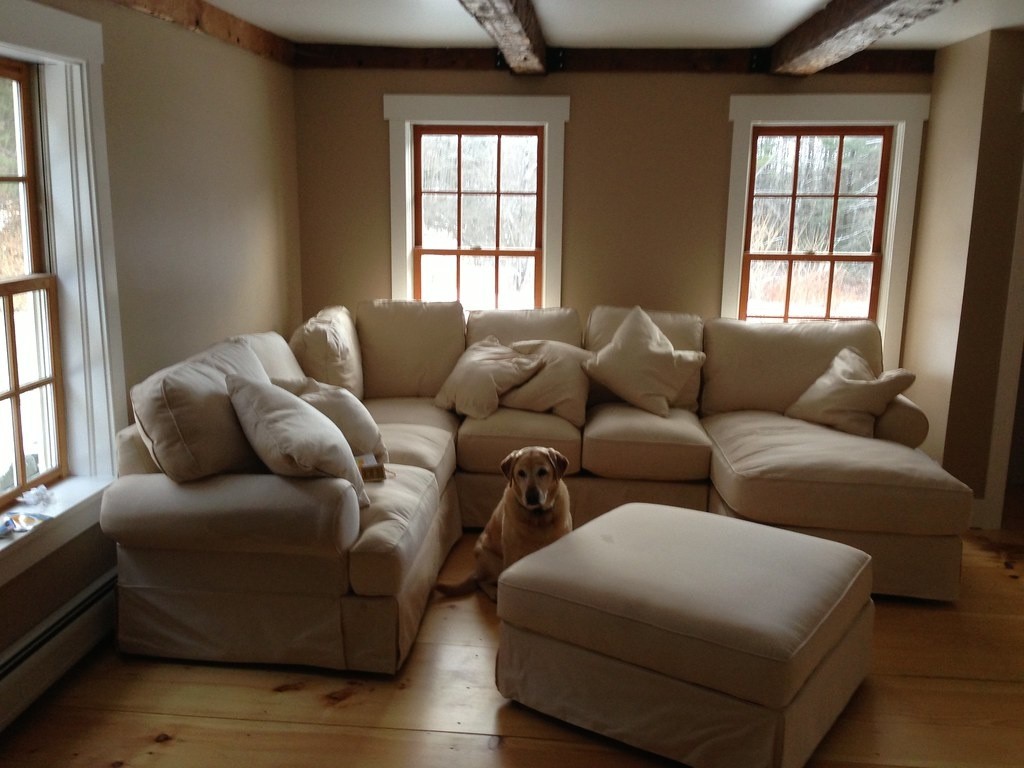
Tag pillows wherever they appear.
[499,341,596,427]
[225,373,371,508]
[269,376,389,465]
[580,305,707,418]
[431,334,544,421]
[784,344,916,438]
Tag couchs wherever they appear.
[700,314,975,608]
[453,307,713,534]
[98,299,467,682]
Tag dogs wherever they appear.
[434,446,573,602]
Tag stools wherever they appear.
[495,501,875,768]
